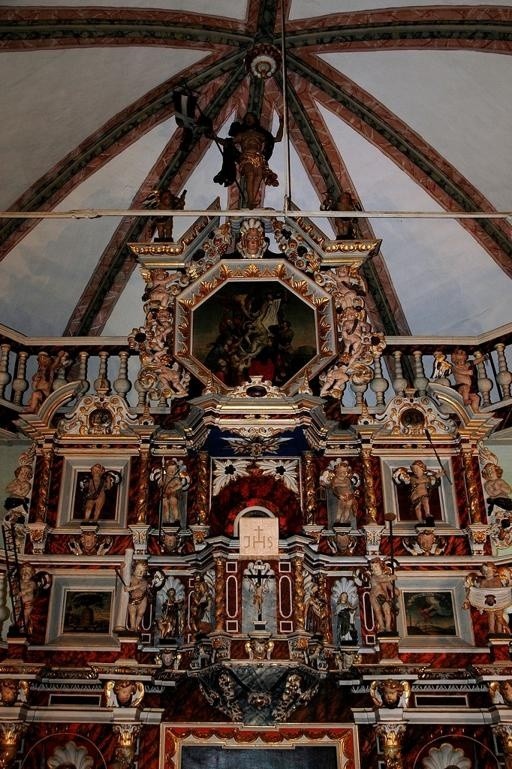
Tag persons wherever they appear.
[323,191,357,244]
[150,188,186,244]
[207,110,284,211]
[3,217,511,769]
